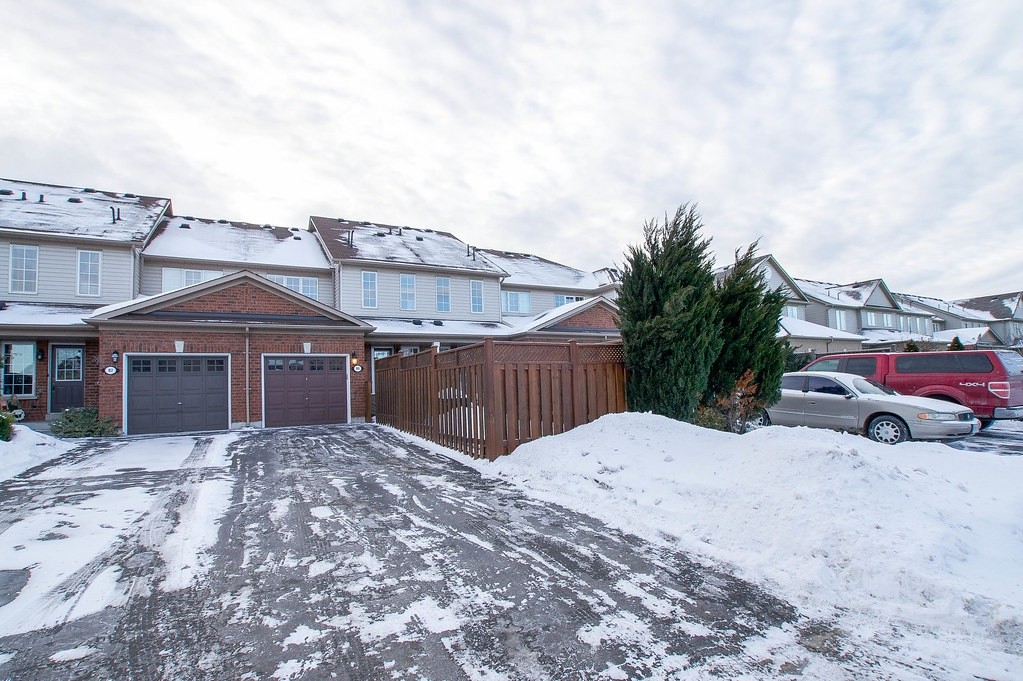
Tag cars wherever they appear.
[750,371,981,445]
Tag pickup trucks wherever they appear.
[798,350,1023,429]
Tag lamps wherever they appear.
[37,348,43,361]
[112,349,119,363]
[351,351,358,365]
